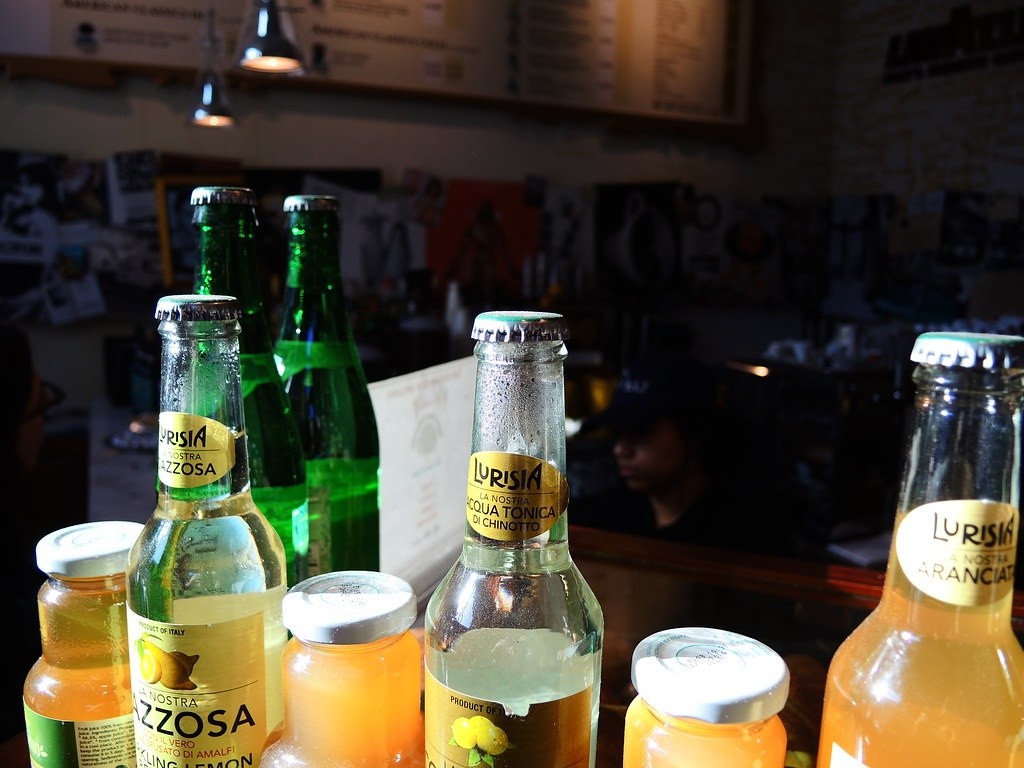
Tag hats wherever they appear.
[584,354,719,430]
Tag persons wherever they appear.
[0,330,50,768]
[584,364,795,559]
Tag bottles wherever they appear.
[423,310,603,768]
[258,570,426,768]
[23,520,159,768]
[275,194,381,577]
[815,331,1024,768]
[622,625,792,768]
[127,294,290,768]
[183,184,310,589]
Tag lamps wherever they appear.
[189,0,235,128]
[231,0,306,73]
